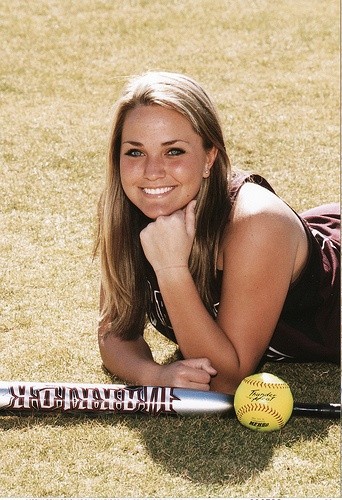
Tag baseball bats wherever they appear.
[0,382,342,420]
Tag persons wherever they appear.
[90,71,342,397]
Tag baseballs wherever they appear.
[234,372,294,431]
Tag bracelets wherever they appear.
[153,263,189,273]
